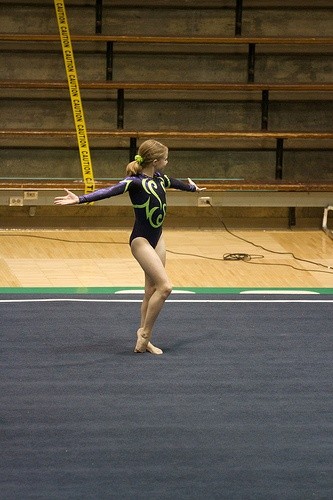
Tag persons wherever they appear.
[54,140,206,356]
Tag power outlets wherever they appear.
[9,197,23,206]
[198,197,211,207]
[23,192,39,199]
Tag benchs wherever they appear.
[0,0,333,230]
